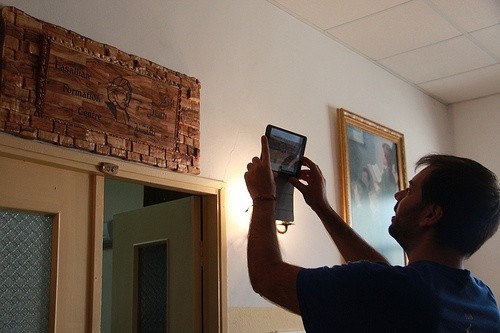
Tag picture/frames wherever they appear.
[337,108,410,267]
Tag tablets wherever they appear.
[260,124,307,177]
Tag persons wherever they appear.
[243,134,500,333]
